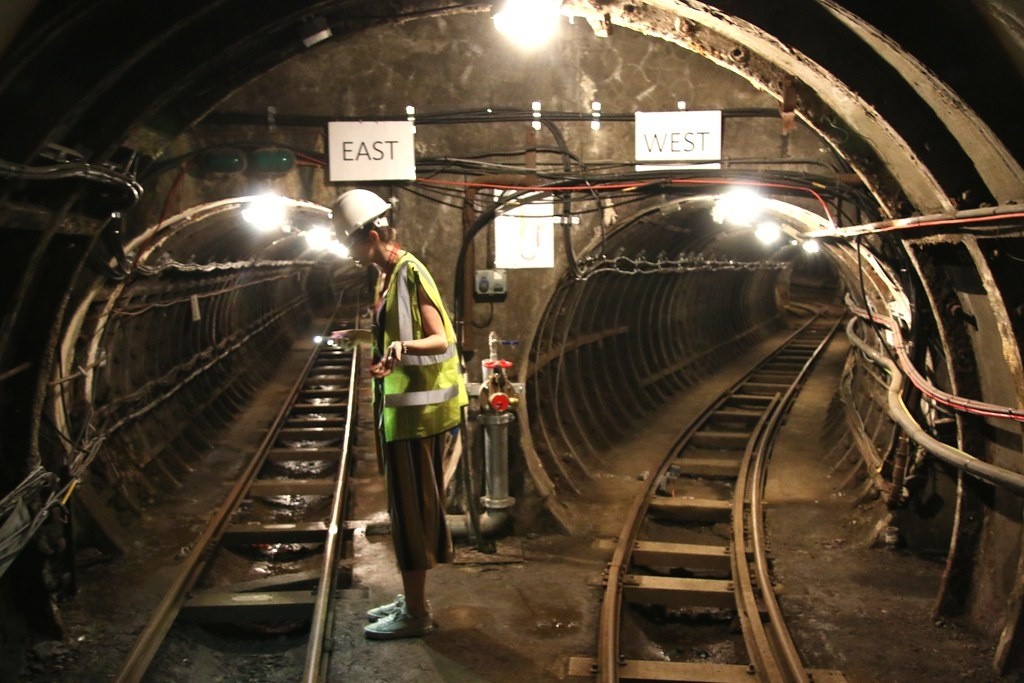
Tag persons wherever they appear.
[330,189,467,640]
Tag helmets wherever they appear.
[332,189,392,243]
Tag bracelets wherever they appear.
[401,341,407,355]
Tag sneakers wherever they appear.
[366,597,433,621]
[363,609,433,639]
